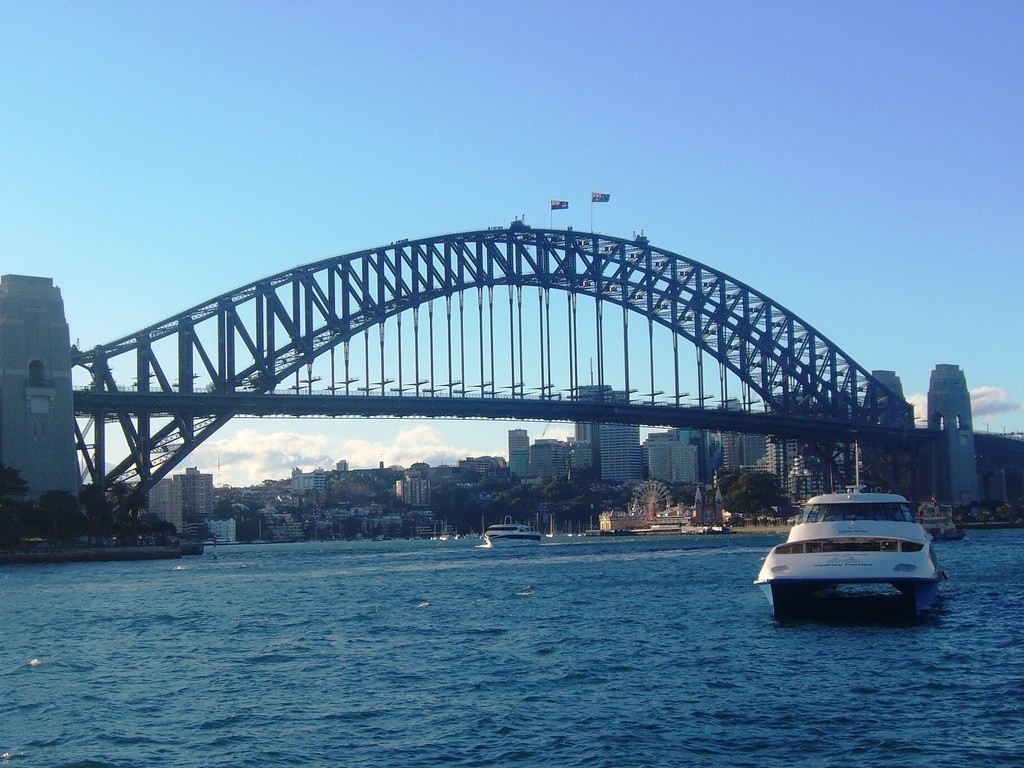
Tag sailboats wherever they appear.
[251,520,271,543]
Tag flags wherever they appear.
[551,200,568,210]
[592,193,611,202]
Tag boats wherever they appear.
[251,538,270,544]
[483,516,541,545]
[753,485,947,623]
[203,537,239,545]
[916,498,965,540]
